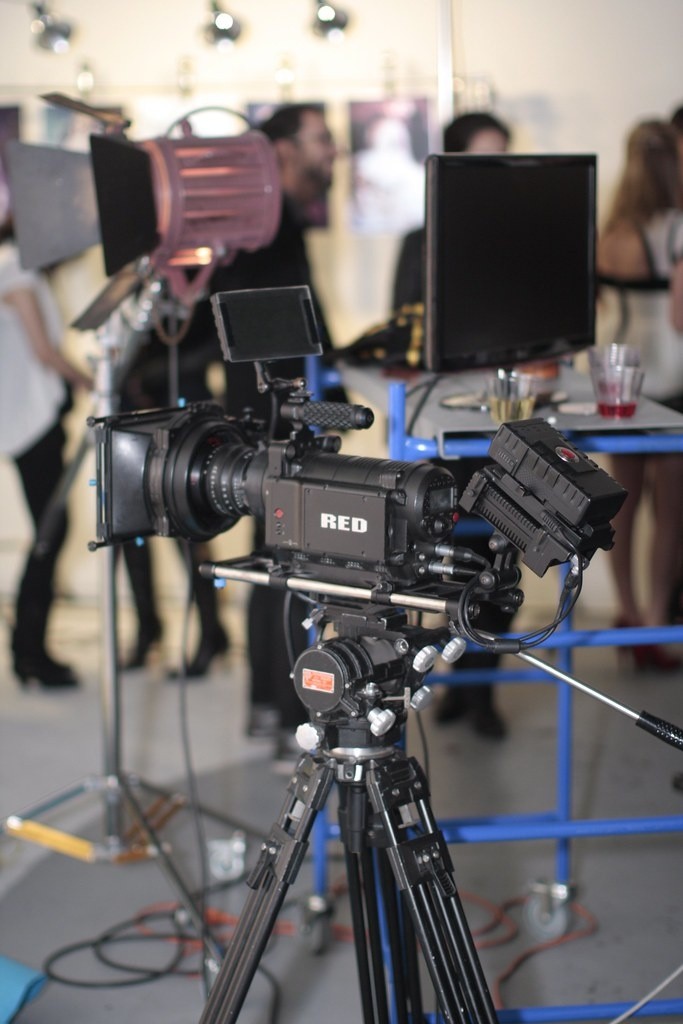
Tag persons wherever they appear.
[104,334,236,688]
[0,207,97,690]
[591,118,683,668]
[666,104,683,623]
[391,111,516,742]
[167,100,355,760]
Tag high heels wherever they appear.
[12,559,77,690]
[119,541,162,671]
[613,616,681,672]
[171,554,233,679]
[431,640,510,742]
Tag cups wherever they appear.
[587,341,642,400]
[487,371,539,423]
[589,365,645,419]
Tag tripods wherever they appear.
[196,591,683,1024]
[0,310,276,968]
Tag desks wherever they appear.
[307,358,682,952]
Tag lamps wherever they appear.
[2,87,284,330]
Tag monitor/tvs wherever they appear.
[425,152,598,411]
